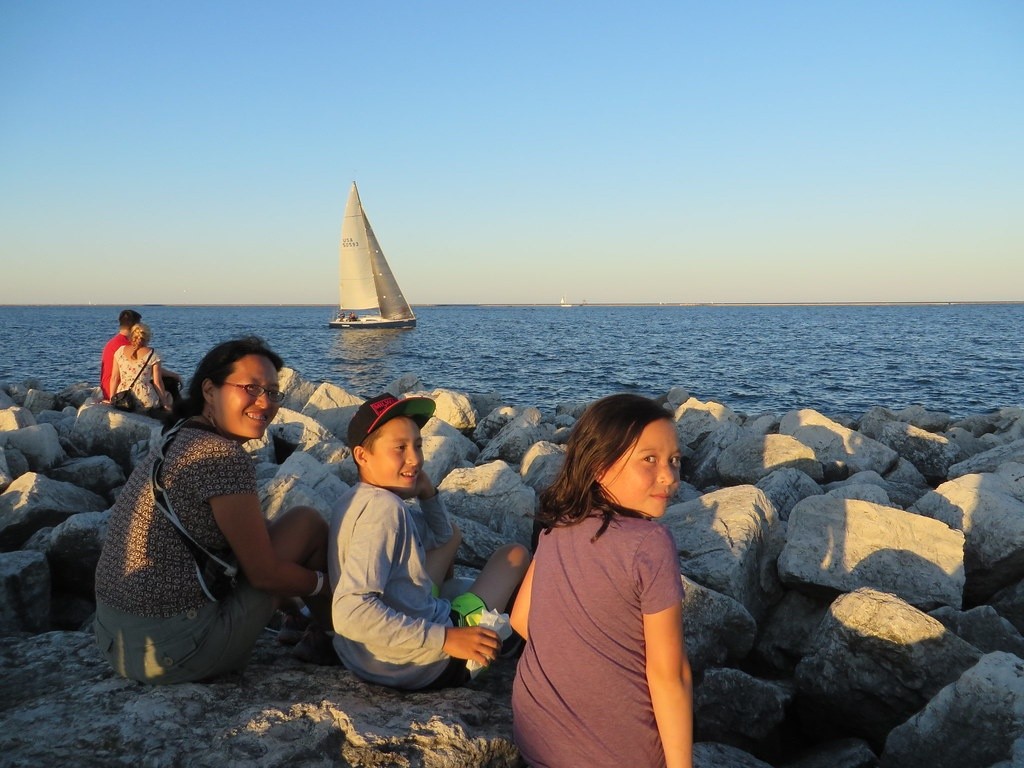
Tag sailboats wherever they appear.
[329,182,417,330]
[560,296,572,307]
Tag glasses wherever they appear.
[223,381,284,403]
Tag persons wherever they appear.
[104,323,183,424]
[337,310,362,326]
[328,392,532,694]
[104,310,185,412]
[89,336,334,688]
[509,394,695,767]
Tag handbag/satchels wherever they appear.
[111,390,137,413]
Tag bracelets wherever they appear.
[308,570,326,599]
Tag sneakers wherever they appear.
[278,616,306,644]
[291,622,344,665]
[500,631,523,659]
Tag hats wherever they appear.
[349,392,436,464]
